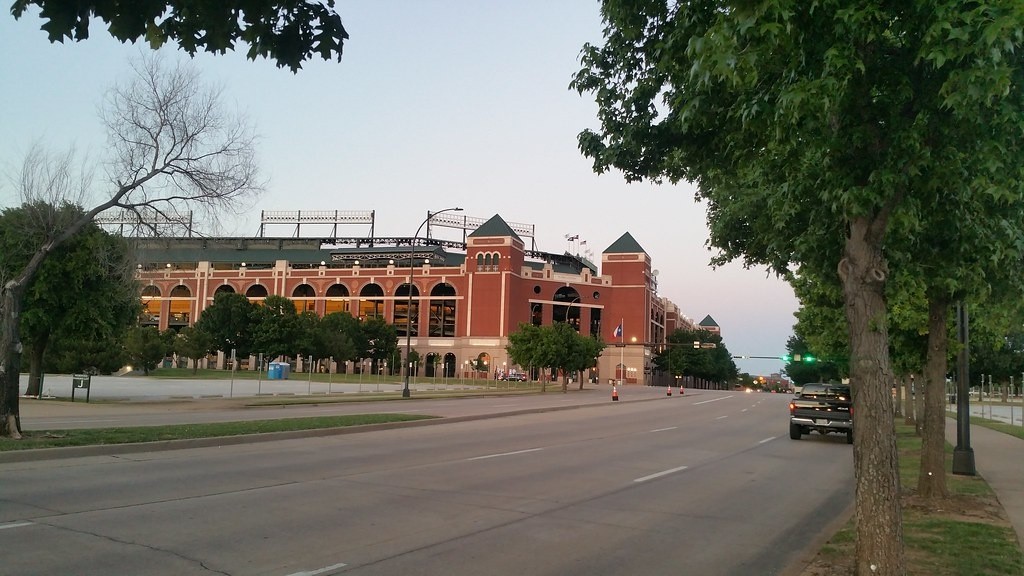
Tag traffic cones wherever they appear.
[611,386,618,401]
[680,385,684,394]
[666,384,672,396]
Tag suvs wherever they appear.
[502,373,527,383]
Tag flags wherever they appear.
[611,320,622,340]
[565,233,594,256]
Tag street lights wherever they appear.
[565,294,589,326]
[402,207,465,398]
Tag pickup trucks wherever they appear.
[789,382,853,444]
